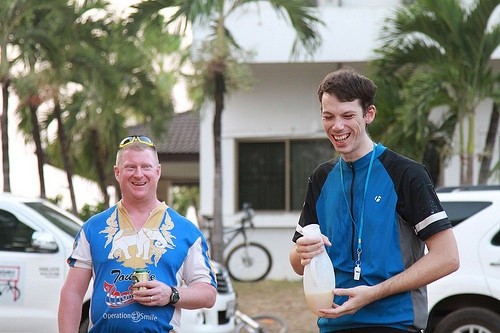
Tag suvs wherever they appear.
[1,192,236,332]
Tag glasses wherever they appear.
[116,135,157,152]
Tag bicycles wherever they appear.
[202,204,273,283]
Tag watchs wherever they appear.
[170,287,180,304]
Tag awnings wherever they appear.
[190,4,498,63]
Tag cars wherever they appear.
[424,186,500,333]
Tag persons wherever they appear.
[290,66,460,333]
[57,136,219,333]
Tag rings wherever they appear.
[150,296,153,301]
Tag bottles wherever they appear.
[301,224,335,317]
